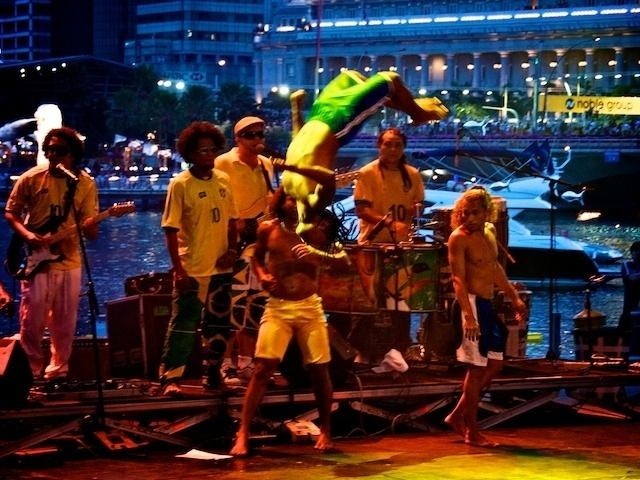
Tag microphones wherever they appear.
[55,162,79,183]
[367,211,392,241]
[455,148,474,159]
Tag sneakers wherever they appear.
[221,364,241,386]
[237,362,254,380]
[164,382,181,396]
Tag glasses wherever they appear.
[198,146,218,156]
[240,131,265,139]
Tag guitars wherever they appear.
[237,167,363,259]
[6,199,136,282]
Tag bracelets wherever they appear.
[227,248,238,254]
[321,250,335,270]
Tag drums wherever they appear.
[317,240,381,316]
[381,241,439,315]
[432,198,508,292]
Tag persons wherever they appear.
[5,127,101,387]
[271,70,449,248]
[228,186,353,456]
[212,117,276,388]
[618,241,640,361]
[146,123,240,397]
[449,184,505,370]
[444,190,529,448]
[0,107,639,183]
[350,128,426,369]
[0,282,12,316]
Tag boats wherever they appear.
[325,188,626,289]
[345,170,602,227]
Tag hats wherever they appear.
[234,116,265,134]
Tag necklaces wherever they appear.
[278,219,301,236]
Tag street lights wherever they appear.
[357,47,408,75]
[213,59,227,122]
[540,36,601,122]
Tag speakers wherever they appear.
[283,326,358,387]
[0,339,32,409]
